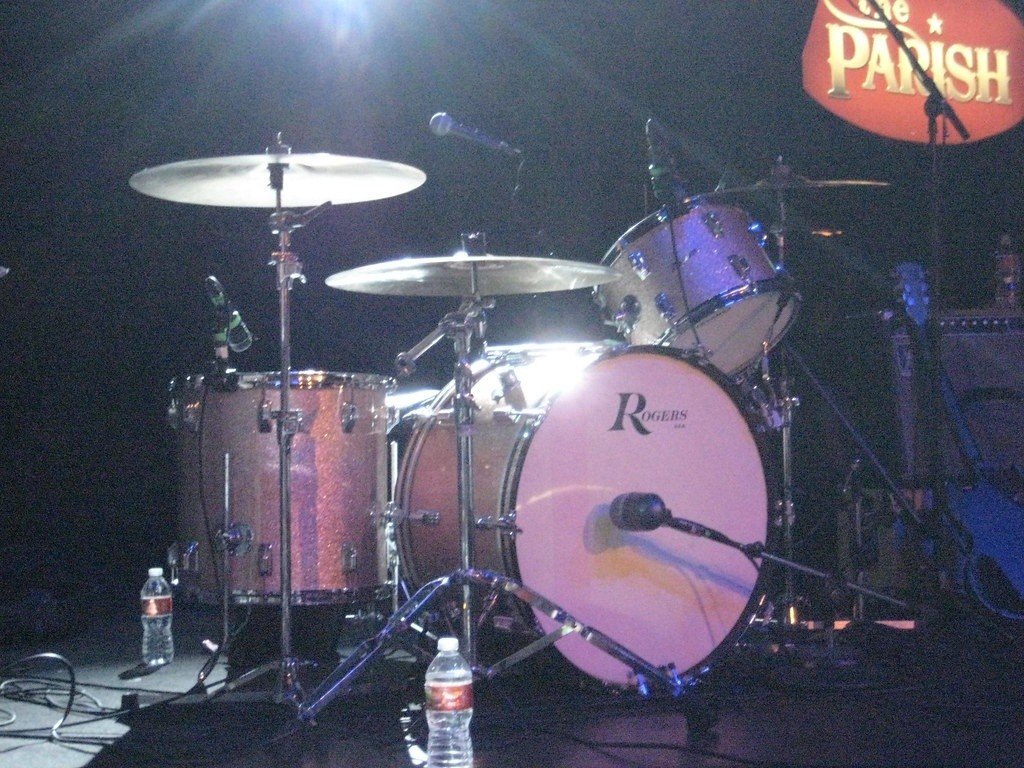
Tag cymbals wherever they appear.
[682,178,892,206]
[126,152,431,212]
[325,249,624,296]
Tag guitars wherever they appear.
[887,259,1024,641]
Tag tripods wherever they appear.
[263,231,724,763]
[175,162,424,727]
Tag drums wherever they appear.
[591,192,804,379]
[389,340,784,703]
[164,366,398,608]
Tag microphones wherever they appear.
[429,112,522,154]
[203,275,252,353]
[645,118,675,200]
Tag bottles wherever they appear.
[139,568,174,667]
[991,233,1021,314]
[423,636,475,768]
